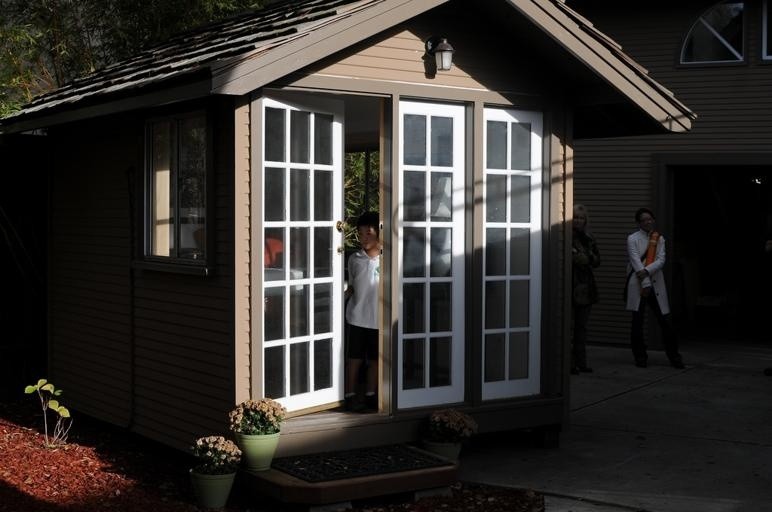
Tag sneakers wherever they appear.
[344,391,378,413]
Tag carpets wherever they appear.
[273,444,455,483]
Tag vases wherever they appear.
[236,433,279,473]
[423,441,463,462]
[187,467,236,507]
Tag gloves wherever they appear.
[639,286,651,298]
[635,268,649,283]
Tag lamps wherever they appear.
[426,33,457,70]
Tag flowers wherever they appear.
[189,435,244,474]
[429,405,480,440]
[227,394,286,433]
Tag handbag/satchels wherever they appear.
[623,287,627,304]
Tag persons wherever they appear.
[625,208,687,369]
[571,203,602,374]
[763,240,772,377]
[345,210,380,400]
